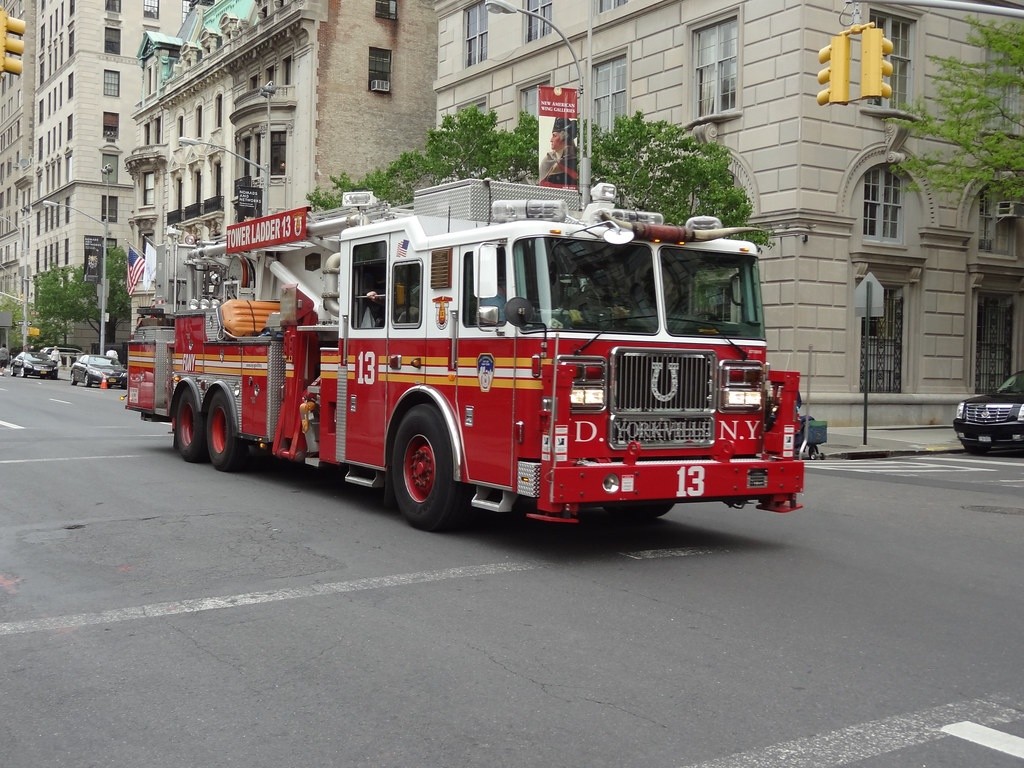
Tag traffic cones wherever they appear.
[100,374,108,389]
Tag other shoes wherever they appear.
[0,373,4,375]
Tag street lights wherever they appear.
[177,87,271,216]
[484,0,594,212]
[39,164,114,355]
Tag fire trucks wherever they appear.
[124,178,807,532]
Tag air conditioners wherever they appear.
[995,201,1024,218]
[371,80,389,92]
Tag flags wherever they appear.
[143,241,157,292]
[126,246,144,297]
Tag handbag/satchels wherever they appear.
[58,360,62,366]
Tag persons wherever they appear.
[106,346,118,363]
[644,265,689,314]
[50,345,62,380]
[367,290,390,309]
[478,273,506,326]
[0,344,9,376]
[542,116,579,189]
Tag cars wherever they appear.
[40,347,87,365]
[70,355,127,388]
[9,351,58,381]
[953,370,1024,454]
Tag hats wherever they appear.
[552,117,578,139]
[54,346,57,348]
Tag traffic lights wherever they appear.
[817,34,851,107]
[861,27,895,100]
